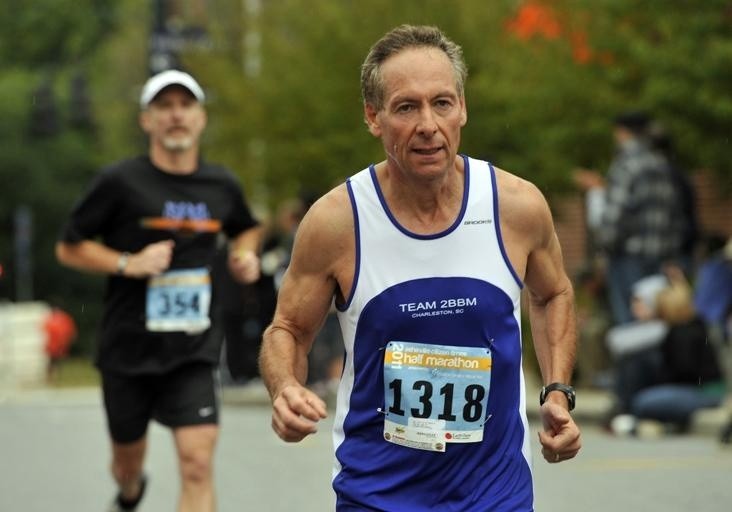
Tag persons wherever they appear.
[600,225,732,447]
[245,190,318,312]
[570,106,686,325]
[253,21,586,511]
[51,62,270,511]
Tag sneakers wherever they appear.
[111,477,146,511]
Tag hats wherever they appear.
[139,67,205,106]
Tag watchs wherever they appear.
[537,381,577,413]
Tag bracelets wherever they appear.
[115,249,132,275]
